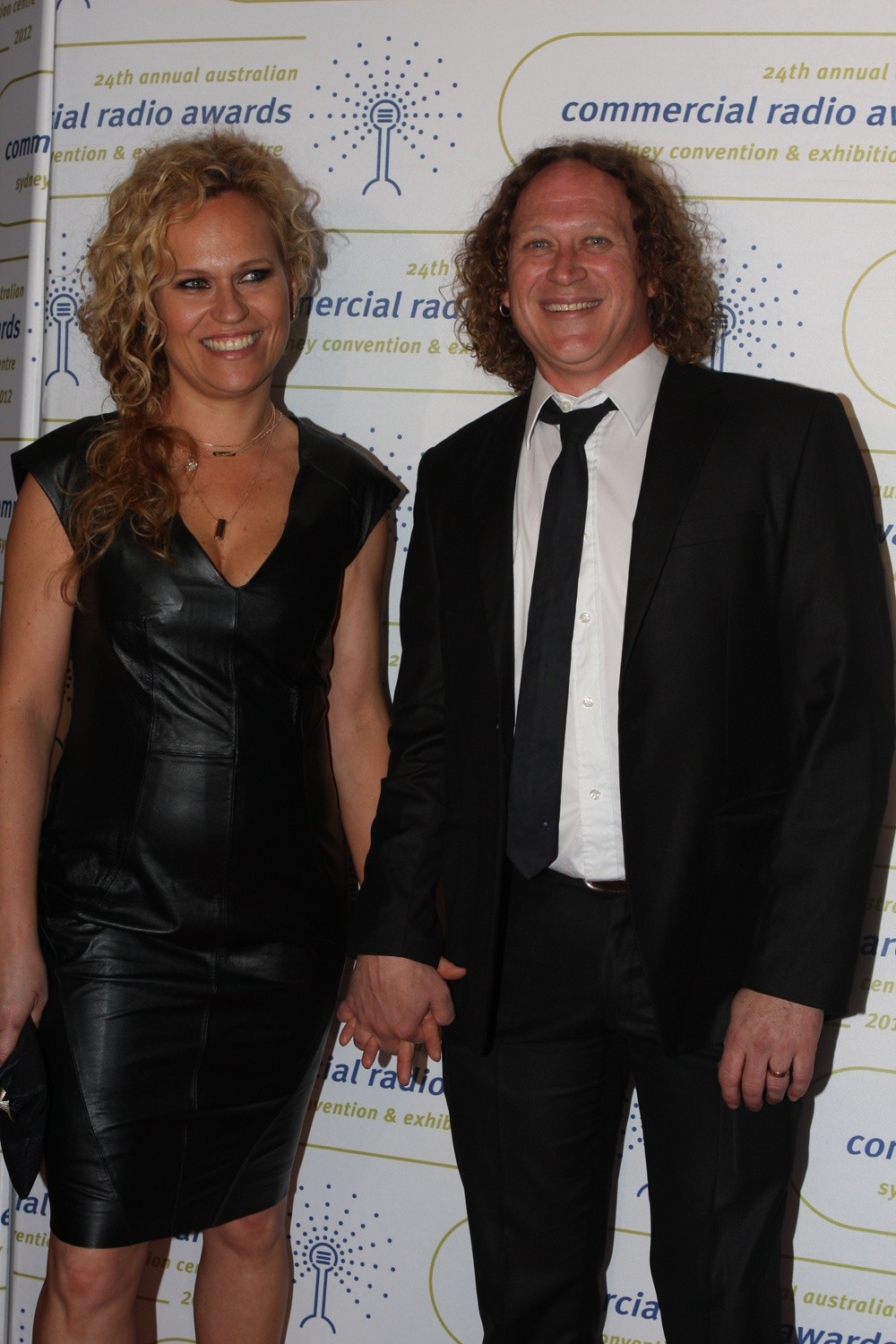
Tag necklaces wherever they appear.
[177,400,283,541]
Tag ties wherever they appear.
[506,396,617,877]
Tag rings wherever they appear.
[768,1065,787,1077]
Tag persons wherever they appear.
[0,133,466,1344]
[345,140,896,1344]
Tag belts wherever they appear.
[537,867,631,892]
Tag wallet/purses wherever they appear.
[0,1017,48,1200]
